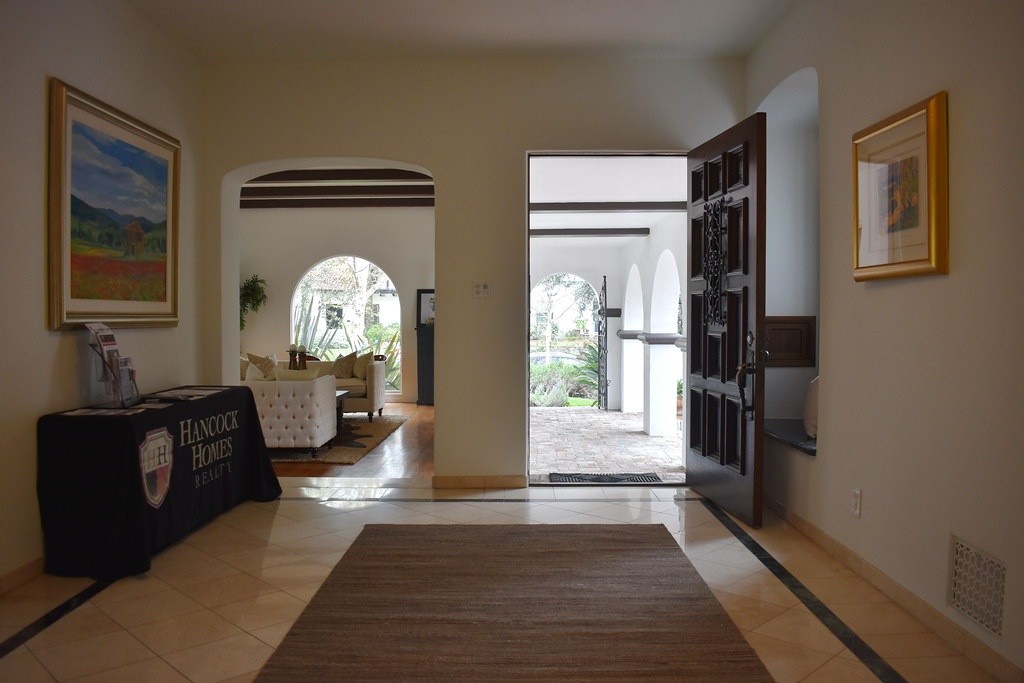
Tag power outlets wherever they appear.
[852,489,861,516]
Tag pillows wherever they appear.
[278,368,319,382]
[240,357,250,379]
[245,362,280,381]
[352,352,375,379]
[804,376,819,440]
[246,353,274,371]
[330,351,358,377]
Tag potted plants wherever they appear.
[677,380,683,415]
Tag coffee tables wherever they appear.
[336,389,350,426]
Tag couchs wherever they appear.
[277,360,387,422]
[241,374,336,456]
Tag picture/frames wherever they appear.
[416,289,436,326]
[852,90,949,281]
[48,76,182,331]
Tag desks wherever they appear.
[37,385,282,581]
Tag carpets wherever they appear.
[253,524,776,683]
[270,413,409,464]
[550,471,662,483]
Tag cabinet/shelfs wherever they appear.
[415,327,433,406]
